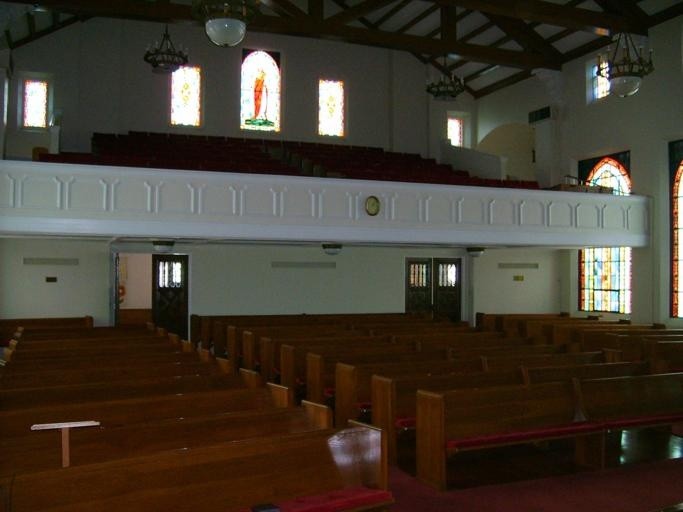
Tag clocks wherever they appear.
[365,196,380,216]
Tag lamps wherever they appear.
[425,56,465,100]
[204,0,248,47]
[145,19,190,74]
[596,24,655,98]
